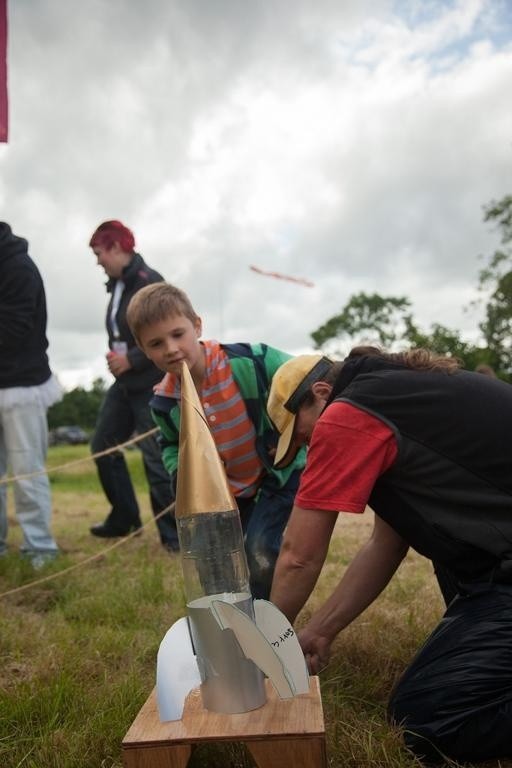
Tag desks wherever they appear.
[121,674,328,768]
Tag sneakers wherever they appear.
[90,515,180,554]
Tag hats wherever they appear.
[264,353,335,471]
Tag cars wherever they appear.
[55,425,90,447]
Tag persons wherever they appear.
[88,219,182,554]
[2,220,58,552]
[264,344,511,768]
[126,278,309,600]
